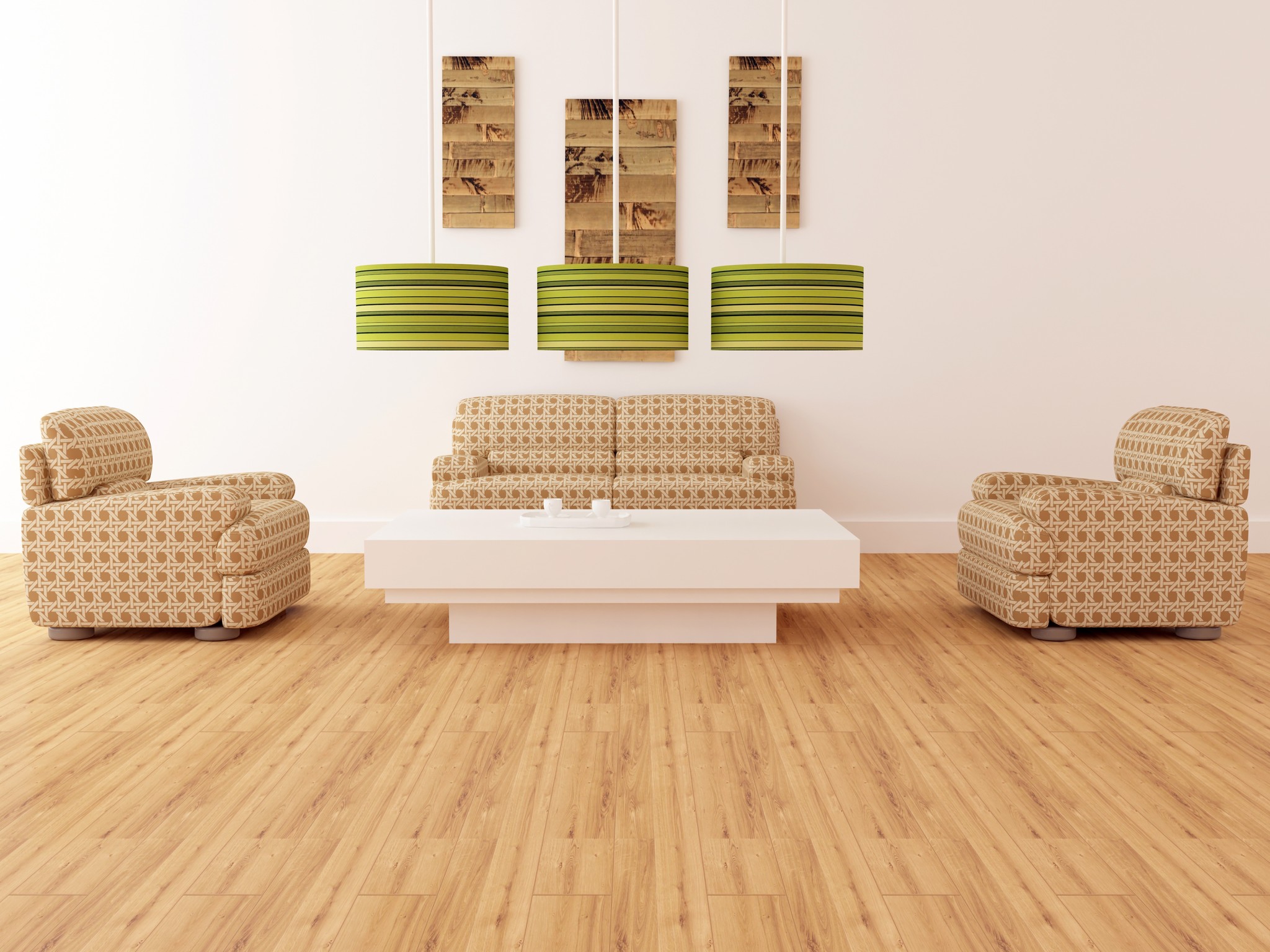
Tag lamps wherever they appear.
[537,0,688,350]
[711,0,864,352]
[355,0,508,351]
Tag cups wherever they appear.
[592,499,612,518]
[544,498,563,517]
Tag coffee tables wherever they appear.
[365,509,860,644]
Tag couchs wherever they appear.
[19,406,310,641]
[958,405,1250,641]
[430,395,796,510]
